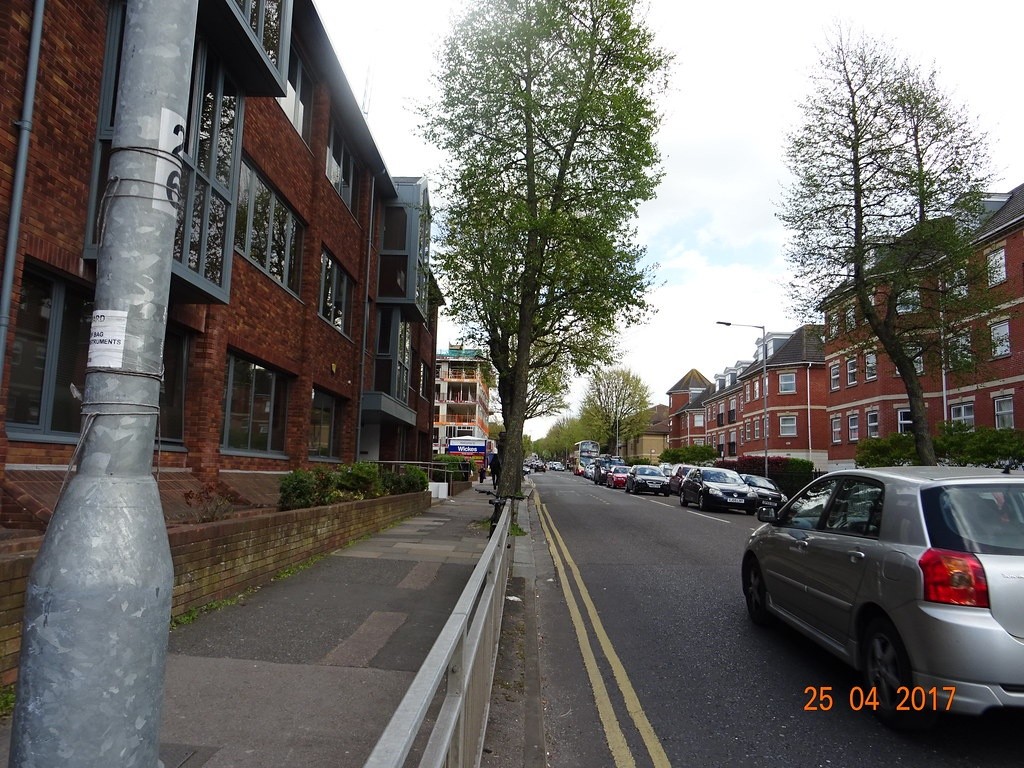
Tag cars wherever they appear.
[669,463,698,495]
[523,456,565,474]
[739,474,789,516]
[568,440,671,496]
[625,464,670,497]
[605,465,631,488]
[678,466,759,515]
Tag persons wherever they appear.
[488,454,501,490]
[478,465,486,483]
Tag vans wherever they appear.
[740,463,1024,733]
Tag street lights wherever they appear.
[717,321,768,478]
